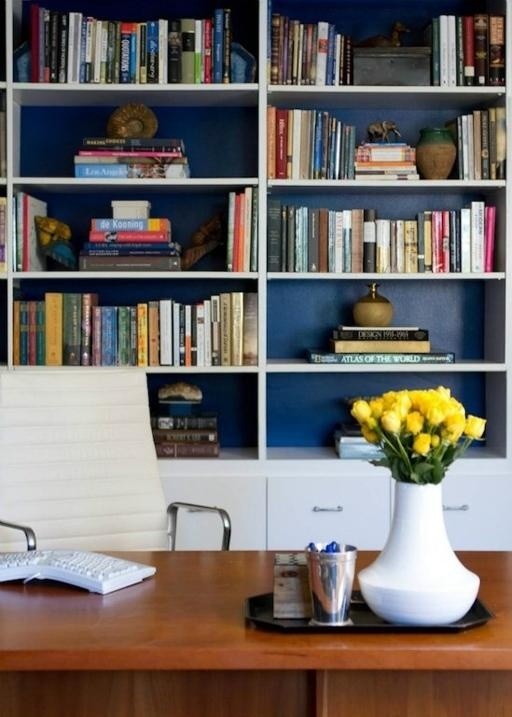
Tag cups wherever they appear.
[304,541,359,625]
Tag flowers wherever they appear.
[348,382,490,483]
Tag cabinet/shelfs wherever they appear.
[392,461,512,552]
[0,1,512,461]
[159,461,268,553]
[267,459,393,552]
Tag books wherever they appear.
[309,323,454,364]
[270,13,353,86]
[79,187,259,273]
[75,137,191,179]
[433,16,505,86]
[333,422,389,459]
[267,200,496,275]
[150,416,222,458]
[456,107,505,180]
[28,6,234,84]
[266,104,422,180]
[13,290,258,367]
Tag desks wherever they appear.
[1,552,512,717]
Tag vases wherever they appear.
[410,123,458,179]
[356,479,482,624]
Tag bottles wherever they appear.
[351,282,393,327]
[415,126,457,179]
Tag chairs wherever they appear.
[0,363,239,554]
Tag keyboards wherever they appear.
[0,550,157,594]
